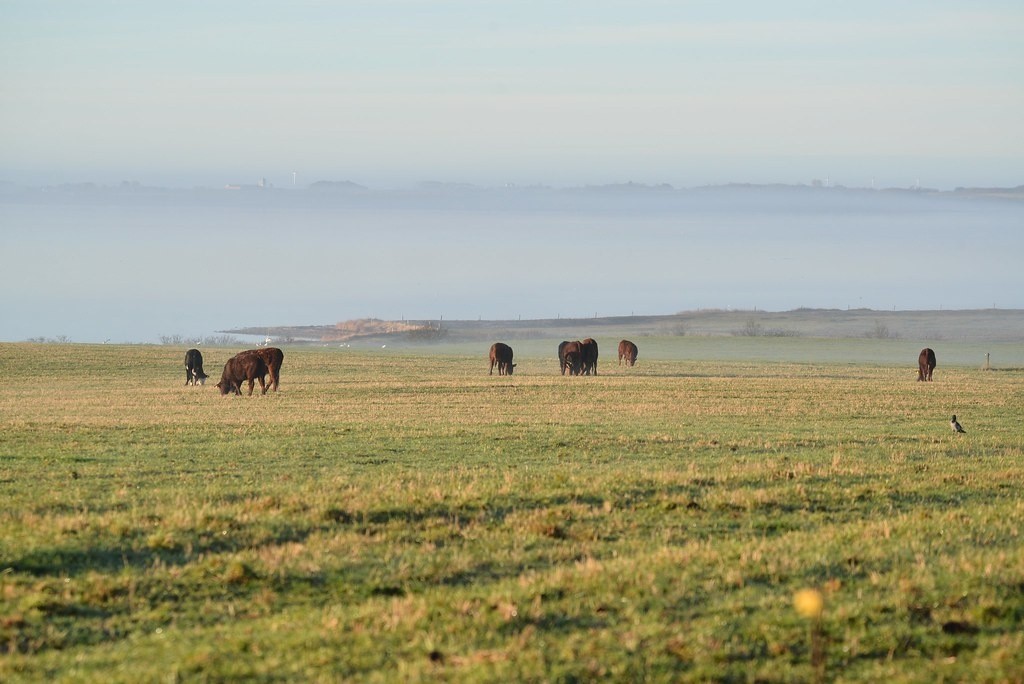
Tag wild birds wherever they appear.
[950,414,967,434]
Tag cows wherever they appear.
[558,338,598,376]
[618,340,638,366]
[916,347,936,381]
[214,347,284,398]
[488,342,517,376]
[184,349,209,386]
